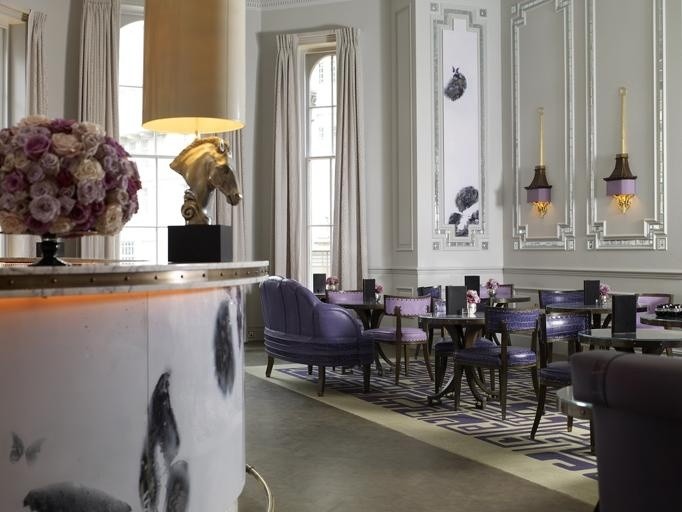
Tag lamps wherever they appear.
[142,0,247,136]
[523,107,552,219]
[602,86,637,215]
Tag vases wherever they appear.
[28,233,72,266]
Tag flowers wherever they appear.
[0,114,142,240]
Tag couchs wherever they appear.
[259,274,374,396]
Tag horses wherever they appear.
[171,137,242,210]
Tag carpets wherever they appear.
[245,355,599,507]
